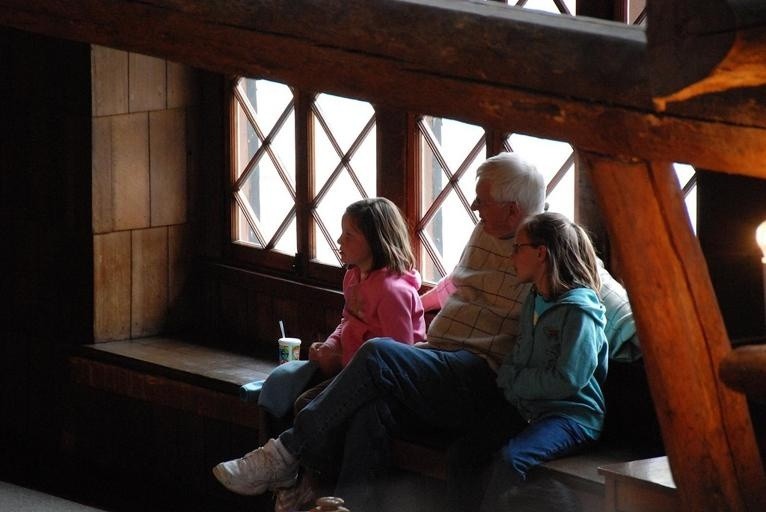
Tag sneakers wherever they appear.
[212,438,315,512]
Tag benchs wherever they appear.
[71,334,623,511]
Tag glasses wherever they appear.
[512,243,537,253]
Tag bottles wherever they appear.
[239,380,265,402]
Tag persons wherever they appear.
[478,212,610,511]
[210,149,642,511]
[256,196,428,436]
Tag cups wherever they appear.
[278,337,302,366]
